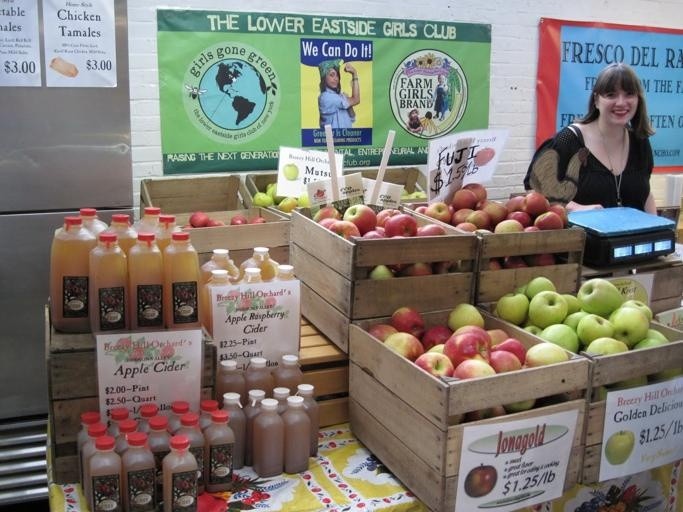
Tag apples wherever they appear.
[606,431,634,465]
[284,164,299,181]
[184,181,681,425]
[464,466,497,498]
[474,148,494,167]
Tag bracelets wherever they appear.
[352,78,358,80]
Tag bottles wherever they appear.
[51,205,301,350]
[76,354,319,512]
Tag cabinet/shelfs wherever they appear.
[45,243,683,512]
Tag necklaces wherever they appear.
[598,117,627,207]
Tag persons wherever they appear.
[420,111,438,135]
[406,107,425,133]
[522,60,659,216]
[431,74,449,121]
[317,59,361,129]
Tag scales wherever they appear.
[566,206,676,269]
